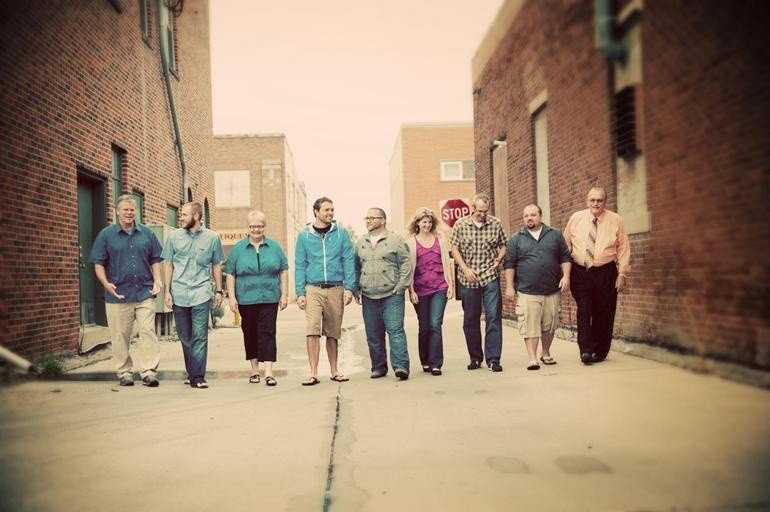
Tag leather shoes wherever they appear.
[582,353,602,362]
[423,365,441,375]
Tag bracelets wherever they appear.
[618,274,627,278]
[216,290,222,293]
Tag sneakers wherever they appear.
[371,369,386,378]
[120,378,133,386]
[142,376,159,386]
[395,368,408,380]
[488,362,501,371]
[469,360,480,369]
[184,378,208,388]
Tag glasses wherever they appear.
[364,216,383,219]
[249,225,264,228]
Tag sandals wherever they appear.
[265,376,276,385]
[249,374,260,382]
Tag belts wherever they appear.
[319,284,336,288]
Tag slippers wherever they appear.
[528,361,539,370]
[540,356,556,364]
[330,375,349,381]
[302,377,320,385]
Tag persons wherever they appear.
[449,192,508,371]
[87,194,164,386]
[505,204,571,370]
[564,186,631,366]
[353,207,412,380]
[405,205,455,376]
[294,197,355,386]
[160,200,225,385]
[226,210,289,386]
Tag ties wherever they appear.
[584,218,597,270]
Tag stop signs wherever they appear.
[438,198,474,231]
